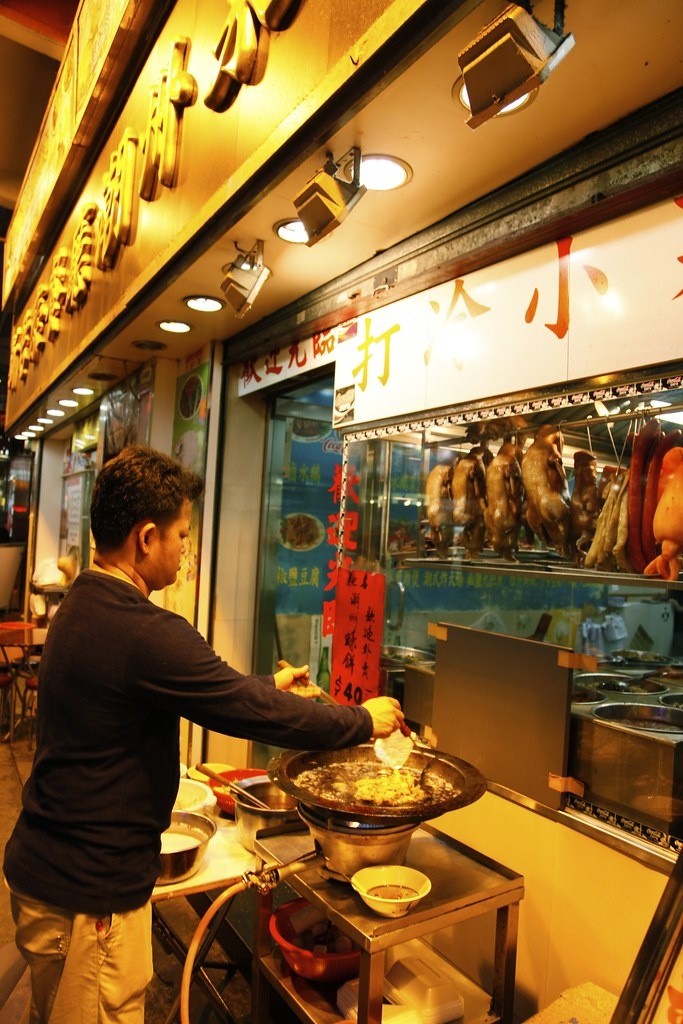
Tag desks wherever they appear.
[0,628,48,742]
[151,804,257,1024]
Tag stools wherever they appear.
[0,658,39,751]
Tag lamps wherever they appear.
[220,242,272,318]
[457,0,576,130]
[292,146,368,248]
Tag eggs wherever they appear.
[335,936,352,952]
[312,923,327,936]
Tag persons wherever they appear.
[3,447,404,1024]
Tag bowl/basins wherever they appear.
[350,866,431,919]
[380,646,436,667]
[154,812,217,886]
[209,769,268,812]
[172,778,217,815]
[230,775,299,853]
[270,896,363,979]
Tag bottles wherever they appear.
[317,648,331,703]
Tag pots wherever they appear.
[264,743,486,825]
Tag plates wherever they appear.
[187,763,236,782]
[277,513,327,551]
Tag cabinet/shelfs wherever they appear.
[204,821,525,1024]
[401,400,683,590]
